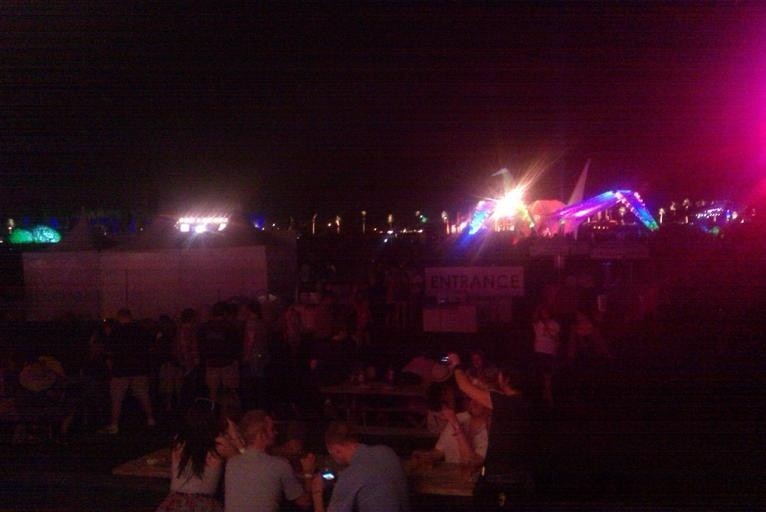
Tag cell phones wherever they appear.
[321,470,337,482]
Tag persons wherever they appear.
[0,223,620,512]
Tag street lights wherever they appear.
[312,214,317,234]
[335,215,341,233]
[387,212,394,231]
[441,210,447,234]
[618,198,704,225]
[361,211,366,233]
[415,210,421,231]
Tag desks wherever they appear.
[111,372,481,498]
